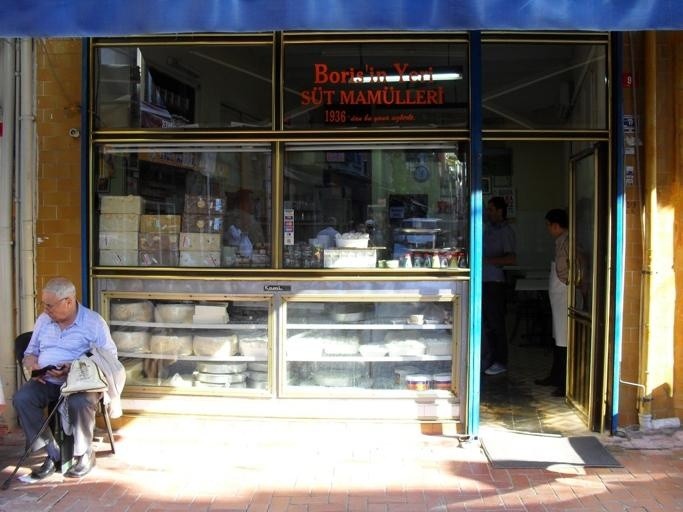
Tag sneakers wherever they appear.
[483,362,507,375]
[534,374,564,396]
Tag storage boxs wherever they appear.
[99,193,226,268]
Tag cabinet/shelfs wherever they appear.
[103,290,461,402]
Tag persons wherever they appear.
[13,277,126,478]
[227,217,243,245]
[313,217,378,249]
[480,196,582,398]
[223,189,264,248]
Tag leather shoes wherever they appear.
[29,455,62,478]
[66,449,98,477]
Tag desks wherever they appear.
[506,264,551,347]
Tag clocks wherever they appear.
[414,165,428,182]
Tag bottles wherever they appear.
[404,250,465,268]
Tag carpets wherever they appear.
[477,428,623,469]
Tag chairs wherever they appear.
[11,331,116,456]
